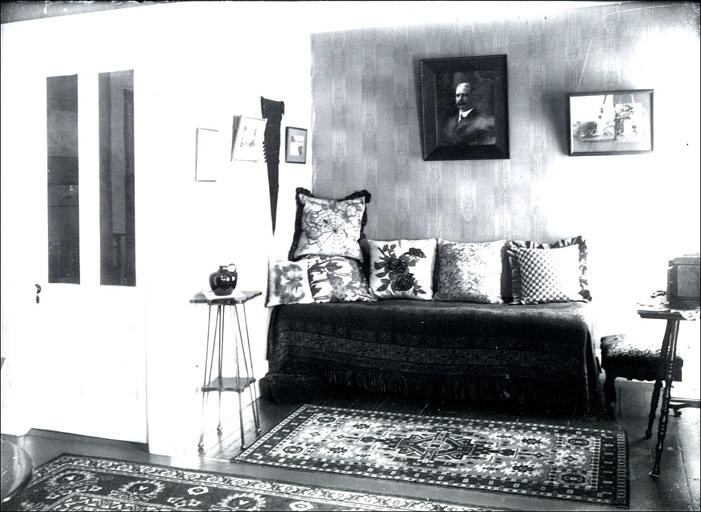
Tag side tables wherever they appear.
[637,299,700,481]
[189,292,263,452]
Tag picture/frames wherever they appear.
[284,126,308,164]
[419,54,510,161]
[231,115,267,162]
[195,127,222,183]
[567,88,654,156]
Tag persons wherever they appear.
[440,82,488,147]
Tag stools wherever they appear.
[600,332,683,417]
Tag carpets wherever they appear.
[1,451,506,512]
[229,403,631,510]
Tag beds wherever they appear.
[259,297,602,421]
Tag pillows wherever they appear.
[299,252,378,304]
[264,257,314,308]
[506,234,592,305]
[288,187,371,262]
[431,238,508,304]
[367,237,437,302]
